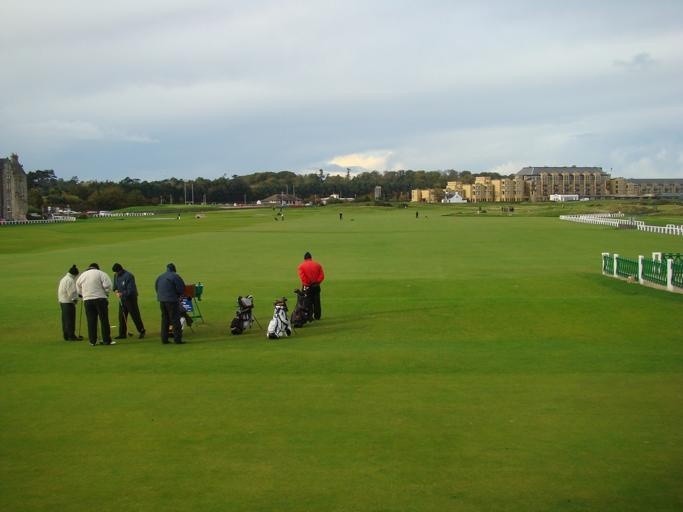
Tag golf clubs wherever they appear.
[79,303,83,338]
[119,298,133,337]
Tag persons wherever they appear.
[56,265,80,341]
[297,252,325,323]
[74,262,114,347]
[111,262,146,340]
[153,263,187,345]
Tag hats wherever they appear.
[303,252,312,260]
[166,263,176,272]
[69,264,79,275]
[89,262,99,269]
[112,263,123,271]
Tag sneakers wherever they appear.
[104,341,117,345]
[89,342,96,347]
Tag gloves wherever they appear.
[73,300,78,304]
[78,294,83,298]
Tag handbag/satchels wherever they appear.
[266,296,291,338]
[230,297,253,334]
[291,289,313,327]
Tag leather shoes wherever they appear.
[176,341,187,346]
[115,335,127,339]
[68,337,84,341]
[162,341,171,343]
[139,330,145,339]
